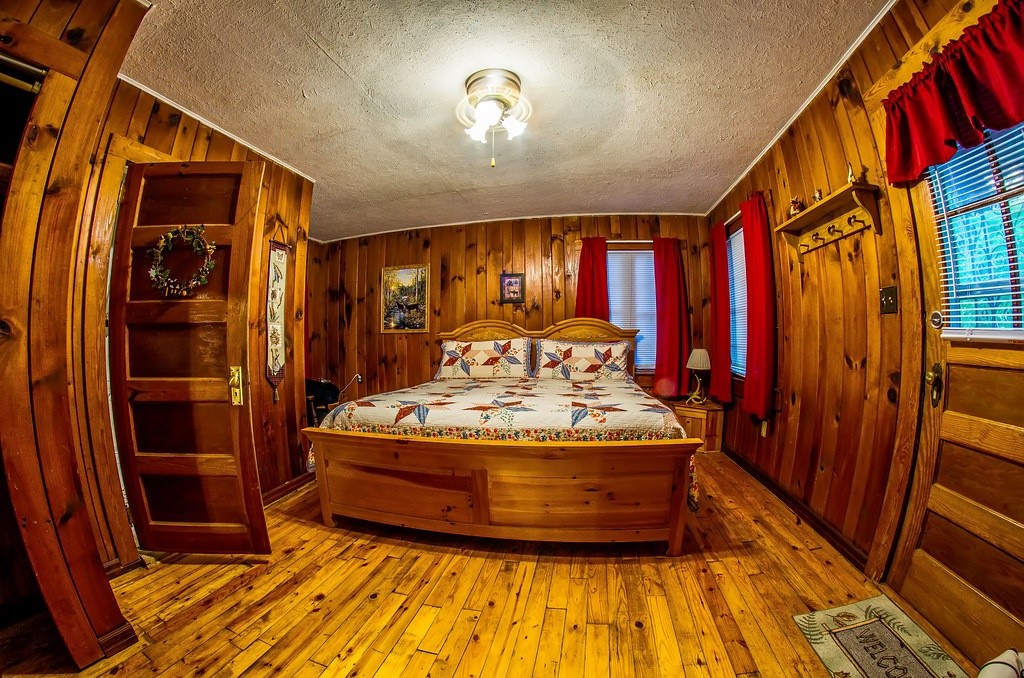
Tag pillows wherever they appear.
[434,337,533,379]
[533,340,634,379]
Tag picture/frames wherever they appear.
[500,272,525,304]
[380,261,431,334]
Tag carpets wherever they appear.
[791,591,970,678]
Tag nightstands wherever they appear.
[670,401,728,457]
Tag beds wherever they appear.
[299,318,702,558]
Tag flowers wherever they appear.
[144,220,216,298]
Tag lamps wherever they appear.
[458,68,533,171]
[686,348,712,405]
